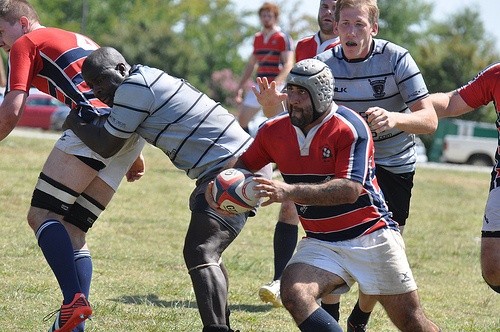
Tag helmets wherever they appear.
[287,59,336,123]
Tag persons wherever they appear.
[61,46,273,332]
[232,0,341,134]
[0,0,145,332]
[205,59,442,332]
[251,0,438,332]
[427,63,500,293]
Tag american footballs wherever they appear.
[212,168,264,215]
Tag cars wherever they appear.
[17,94,64,130]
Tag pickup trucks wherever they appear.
[443,134,498,166]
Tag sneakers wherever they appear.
[43,292,92,332]
[259,280,284,307]
